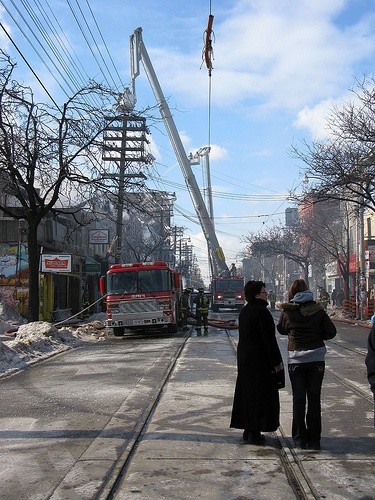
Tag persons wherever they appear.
[229,263,237,279]
[267,290,276,310]
[277,279,337,450]
[320,287,344,313]
[193,288,210,336]
[229,280,285,446]
[180,288,192,330]
[365,320,375,425]
[82,290,89,318]
[359,284,375,308]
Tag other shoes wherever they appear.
[244,433,266,444]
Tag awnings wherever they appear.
[84,256,108,275]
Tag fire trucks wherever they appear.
[119,25,247,312]
[99,261,186,337]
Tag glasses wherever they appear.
[260,290,267,294]
